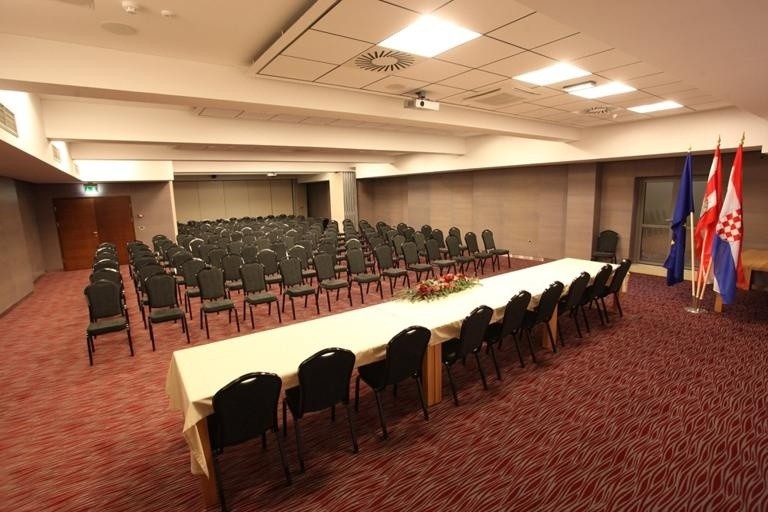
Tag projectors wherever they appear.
[405,99,439,111]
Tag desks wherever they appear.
[733,245,768,289]
[164,253,637,509]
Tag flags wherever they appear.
[691,136,725,285]
[660,144,696,287]
[708,131,750,309]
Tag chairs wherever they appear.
[242,260,282,329]
[198,372,300,511]
[439,255,635,411]
[279,256,319,318]
[135,264,174,330]
[314,253,354,313]
[257,249,283,295]
[197,267,240,340]
[75,210,511,283]
[353,322,435,448]
[345,248,386,303]
[84,278,134,367]
[287,245,324,293]
[183,260,209,320]
[145,273,190,352]
[282,342,359,477]
[221,254,247,312]
[374,244,411,294]
[593,226,620,269]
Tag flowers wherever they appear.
[392,264,485,304]
